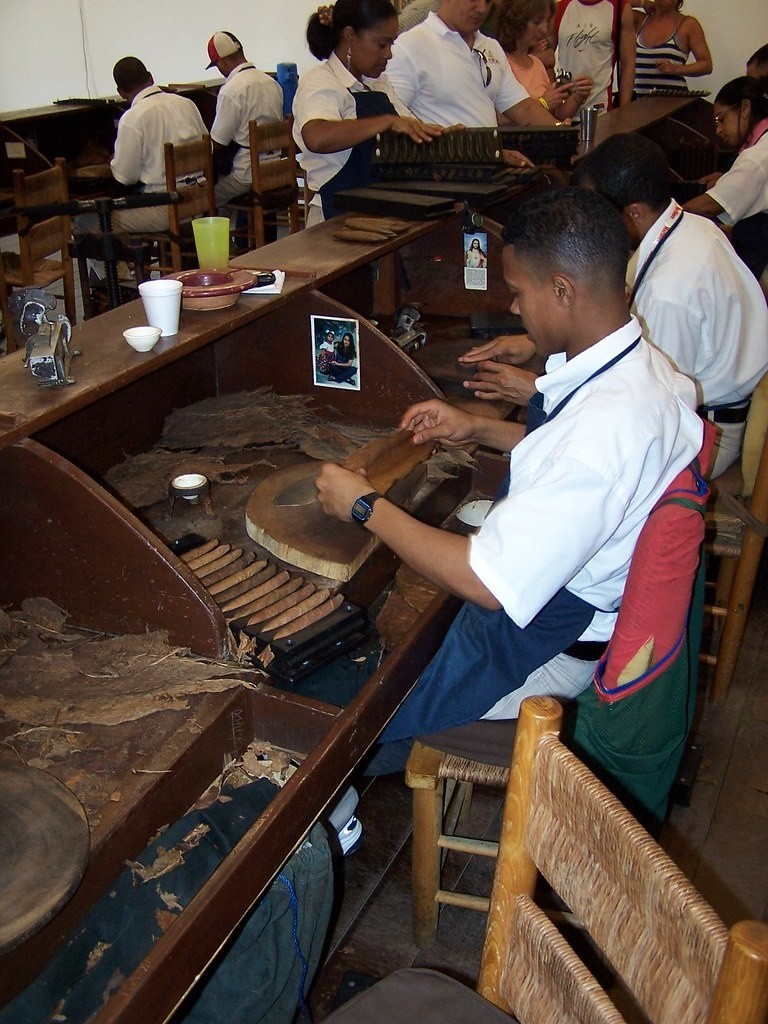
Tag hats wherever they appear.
[205,31,242,69]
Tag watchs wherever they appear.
[351,492,383,527]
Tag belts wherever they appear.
[561,641,610,661]
[695,401,752,423]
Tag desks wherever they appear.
[0,72,739,1024]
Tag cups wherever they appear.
[579,107,598,141]
[138,280,183,337]
[192,217,230,269]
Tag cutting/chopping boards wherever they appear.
[412,339,516,396]
[246,458,427,583]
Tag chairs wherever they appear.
[219,112,300,252]
[697,369,768,710]
[0,156,78,354]
[402,418,716,951]
[116,134,217,273]
[320,693,768,1024]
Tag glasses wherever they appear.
[473,48,491,87]
[714,105,735,124]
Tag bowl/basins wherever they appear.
[123,326,162,352]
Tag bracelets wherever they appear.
[538,97,549,111]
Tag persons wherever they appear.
[292,0,768,241]
[319,329,357,386]
[206,31,283,207]
[466,238,486,268]
[457,150,768,480]
[74,56,214,307]
[317,184,704,856]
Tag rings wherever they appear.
[562,99,565,105]
[588,86,592,90]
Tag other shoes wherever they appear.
[345,378,355,385]
[339,814,366,856]
[328,377,334,381]
